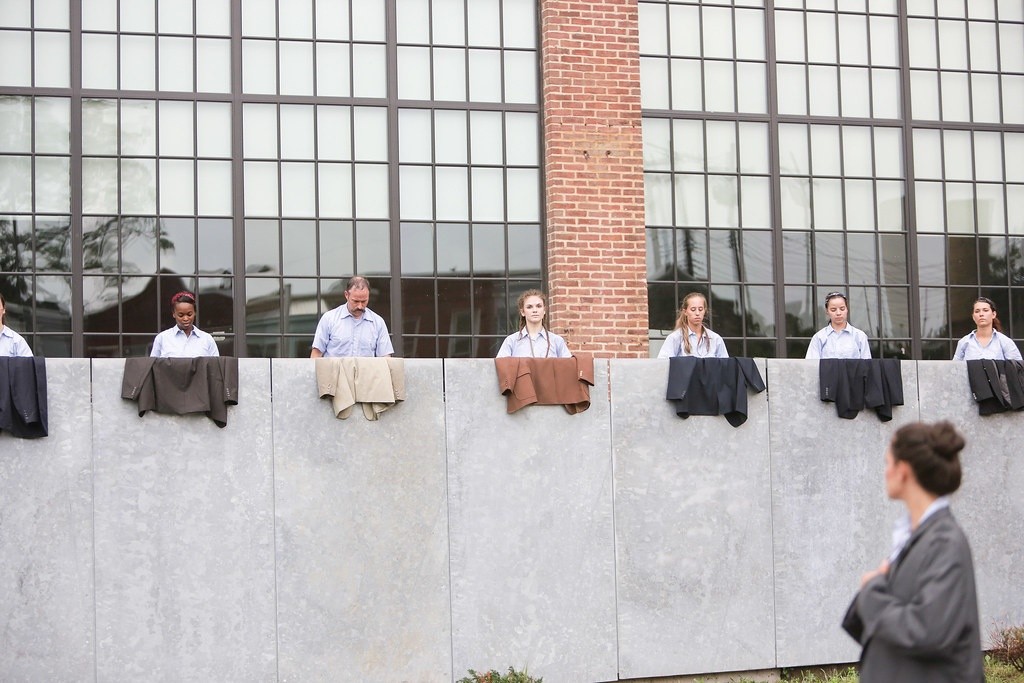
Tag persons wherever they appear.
[150,289,219,358]
[0,294,35,357]
[657,293,730,358]
[952,297,1023,361]
[495,289,573,358]
[310,277,395,359]
[804,291,872,360]
[841,421,988,683]
